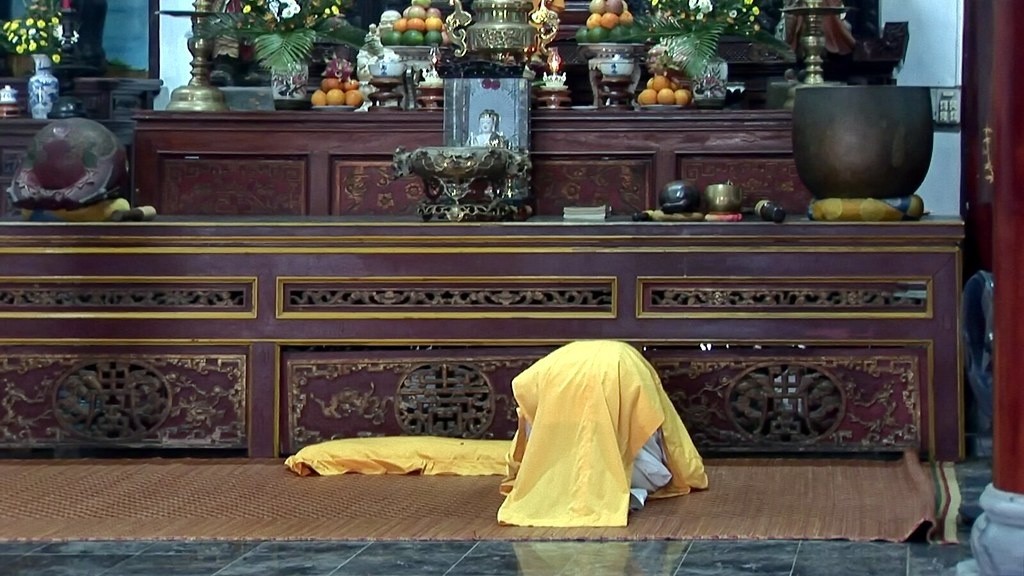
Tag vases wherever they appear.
[681,45,728,104]
[27,50,62,117]
[272,48,309,108]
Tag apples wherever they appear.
[321,78,359,92]
[403,0,441,19]
[589,0,628,16]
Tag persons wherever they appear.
[356,10,404,107]
[501,340,709,529]
[463,111,506,200]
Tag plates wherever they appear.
[382,45,448,54]
[577,41,646,53]
[640,104,682,110]
[419,81,442,88]
[310,104,357,112]
[540,85,569,91]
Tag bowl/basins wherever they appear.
[599,54,635,76]
[368,63,407,77]
[703,184,743,215]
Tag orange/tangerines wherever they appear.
[576,12,642,42]
[638,76,692,105]
[312,88,363,107]
[380,18,444,46]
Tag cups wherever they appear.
[0,85,18,104]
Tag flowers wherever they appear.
[0,0,80,54]
[594,0,789,61]
[188,1,382,70]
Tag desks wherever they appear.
[0,211,972,464]
[128,112,806,216]
